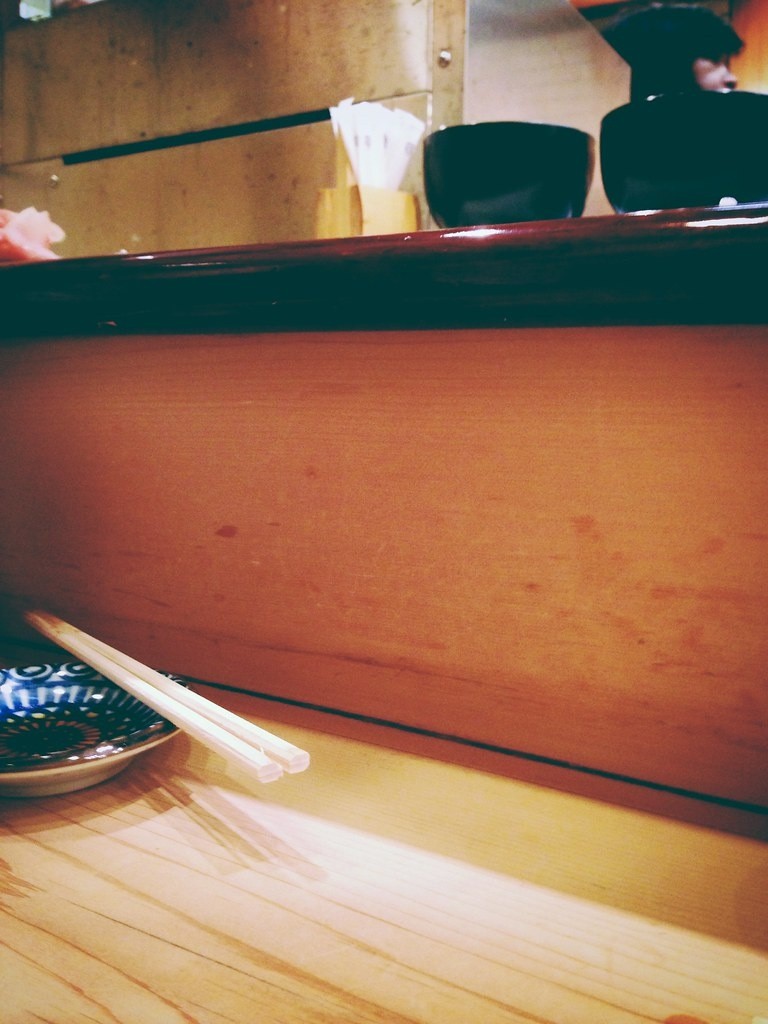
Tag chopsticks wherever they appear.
[11,602,312,786]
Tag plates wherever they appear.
[0,662,196,796]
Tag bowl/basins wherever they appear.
[421,121,597,231]
[600,92,767,214]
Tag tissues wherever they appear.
[315,96,426,242]
